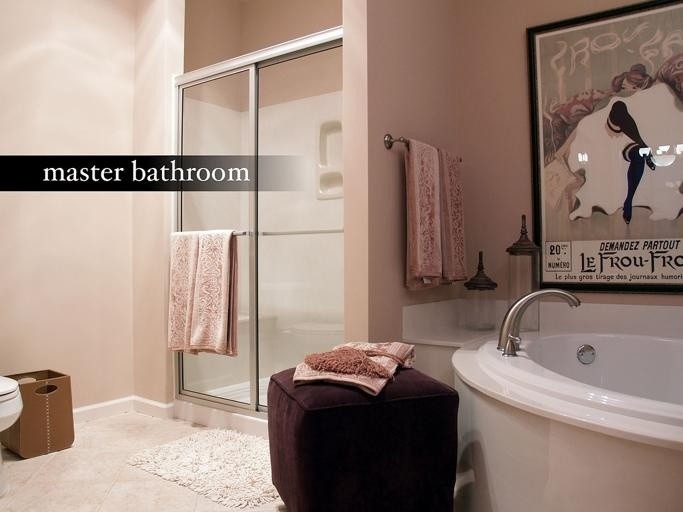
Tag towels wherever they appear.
[166,229,238,359]
[404,138,467,292]
[293,341,416,398]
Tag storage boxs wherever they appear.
[3,369,74,459]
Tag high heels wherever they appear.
[623,200,631,225]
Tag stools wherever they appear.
[267,368,459,512]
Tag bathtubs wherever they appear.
[183,309,278,392]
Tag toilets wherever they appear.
[0,376,24,431]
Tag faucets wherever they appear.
[498,287,582,357]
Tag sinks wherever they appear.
[452,322,683,512]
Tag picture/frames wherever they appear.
[526,0,683,294]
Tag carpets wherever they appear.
[126,428,281,510]
